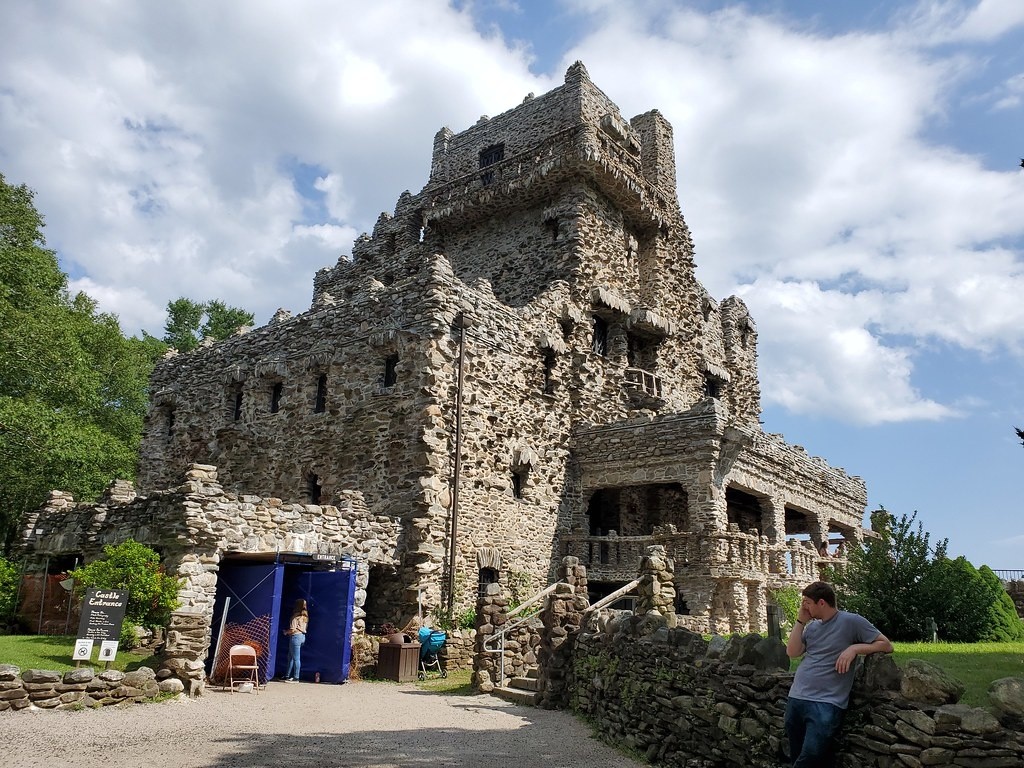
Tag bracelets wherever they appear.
[797,619,807,626]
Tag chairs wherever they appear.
[223,645,260,696]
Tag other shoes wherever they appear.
[278,676,288,681]
[285,676,299,684]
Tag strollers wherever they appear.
[417,625,450,681]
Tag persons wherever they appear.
[819,541,833,571]
[784,582,894,768]
[278,598,309,683]
[837,541,849,557]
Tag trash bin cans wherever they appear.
[378,633,421,683]
